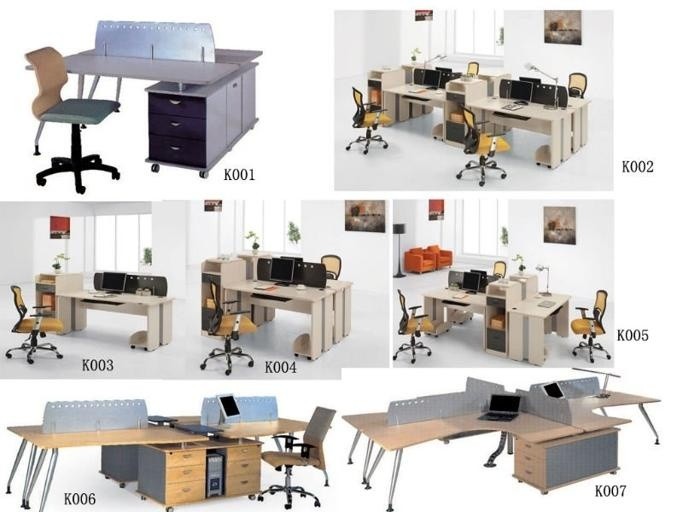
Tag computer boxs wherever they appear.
[205,451,226,498]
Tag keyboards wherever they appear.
[494,112,530,121]
[536,300,556,308]
[81,298,122,307]
[249,292,290,302]
[402,95,428,102]
[442,300,471,307]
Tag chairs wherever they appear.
[25,47,120,194]
[346,87,392,154]
[200,282,253,375]
[249,408,336,510]
[456,104,509,187]
[393,289,431,363]
[424,245,452,271]
[571,290,611,363]
[404,248,436,274]
[6,286,64,364]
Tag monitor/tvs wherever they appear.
[280,256,303,262]
[217,394,243,425]
[540,381,566,400]
[436,66,453,71]
[461,272,482,296]
[509,80,533,106]
[100,271,128,294]
[422,69,442,90]
[471,269,490,288]
[268,258,295,287]
[520,76,541,83]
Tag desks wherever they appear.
[342,368,661,511]
[368,62,591,171]
[26,21,262,178]
[36,274,175,352]
[423,261,570,367]
[6,407,331,512]
[202,255,353,361]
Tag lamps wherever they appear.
[393,224,406,277]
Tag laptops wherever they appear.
[475,393,522,423]
